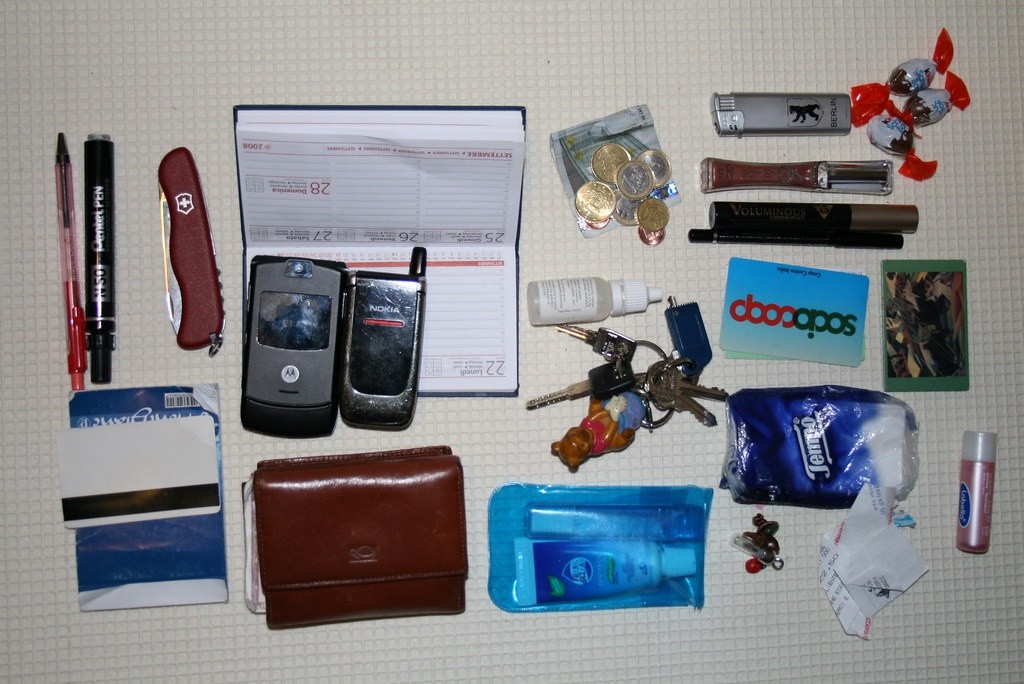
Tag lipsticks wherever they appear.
[700,158,893,196]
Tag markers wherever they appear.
[82,135,117,385]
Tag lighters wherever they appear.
[711,92,851,138]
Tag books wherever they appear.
[232,103,526,397]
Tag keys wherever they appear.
[648,361,729,427]
[557,323,637,363]
[665,295,712,374]
[527,361,635,411]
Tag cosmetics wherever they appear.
[956,431,998,554]
[689,202,919,250]
[514,504,703,607]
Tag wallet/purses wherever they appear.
[253,445,468,630]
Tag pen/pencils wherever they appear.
[54,131,88,392]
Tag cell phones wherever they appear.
[339,247,427,431]
[240,255,349,439]
